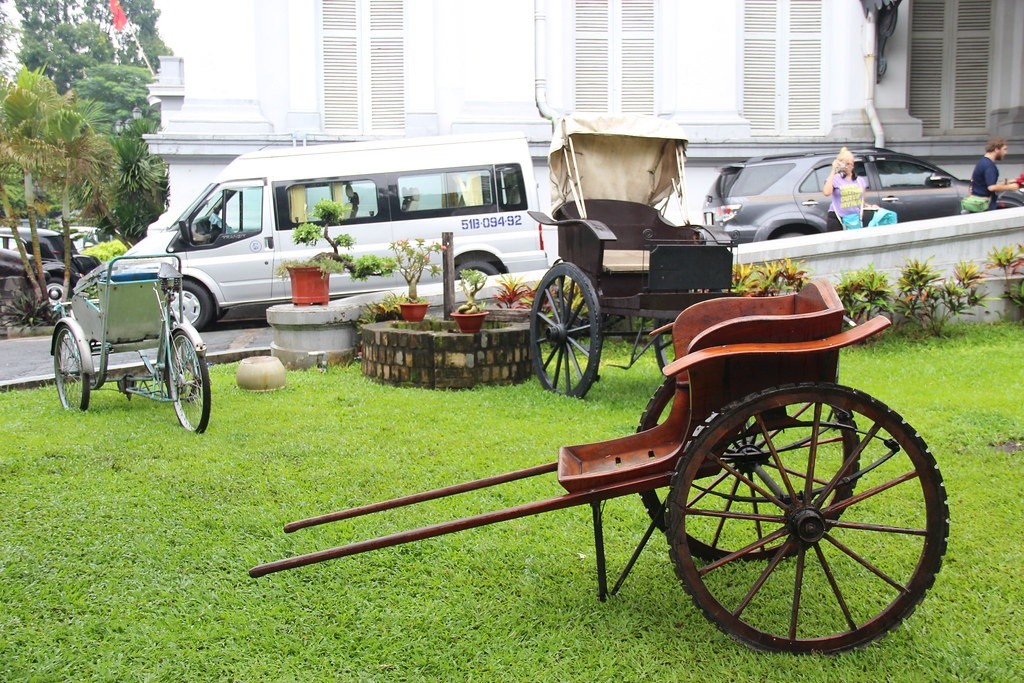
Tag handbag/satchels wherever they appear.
[842,214,863,230]
[960,193,992,213]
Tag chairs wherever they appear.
[342,184,358,217]
[402,186,419,211]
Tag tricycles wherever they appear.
[50,254,213,434]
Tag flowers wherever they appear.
[387,238,449,302]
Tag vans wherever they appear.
[119,132,549,331]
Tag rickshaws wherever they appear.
[526,111,742,400]
[249,278,950,658]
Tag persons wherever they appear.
[824,147,866,232]
[971,137,1024,212]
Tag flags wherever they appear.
[109,0,126,31]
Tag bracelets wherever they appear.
[1004,178,1007,185]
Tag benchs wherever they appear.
[552,199,705,294]
[673,278,843,434]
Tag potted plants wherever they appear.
[450,269,489,334]
[274,200,397,308]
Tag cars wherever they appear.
[0,219,108,325]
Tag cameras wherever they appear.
[838,161,845,169]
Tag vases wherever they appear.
[399,302,431,320]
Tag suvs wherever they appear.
[702,146,1024,249]
